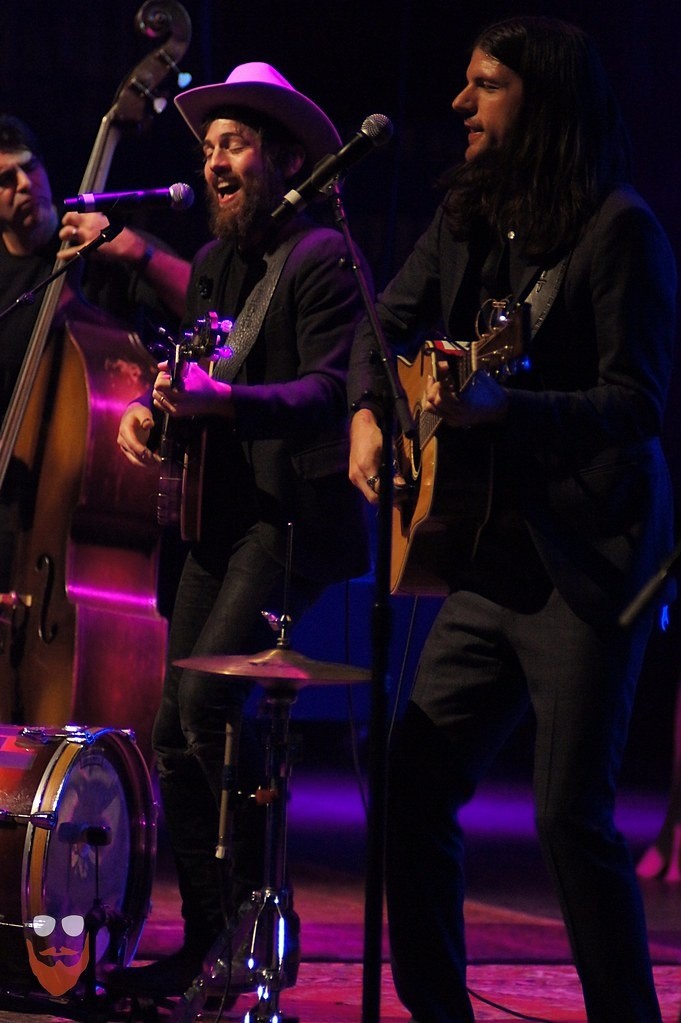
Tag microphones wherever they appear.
[63,183,194,213]
[270,114,393,219]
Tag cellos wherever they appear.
[0,0,198,768]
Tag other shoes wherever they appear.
[195,923,300,994]
[112,944,239,1011]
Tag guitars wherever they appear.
[389,287,531,596]
[155,310,235,542]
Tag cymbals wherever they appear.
[171,647,375,681]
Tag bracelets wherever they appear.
[139,241,155,273]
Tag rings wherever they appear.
[367,474,379,488]
[159,396,165,403]
[71,228,77,239]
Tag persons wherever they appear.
[347,26,681,1023]
[54,63,379,1023]
[0,111,193,685]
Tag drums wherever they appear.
[0,724,159,1014]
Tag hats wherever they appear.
[173,61,345,198]
